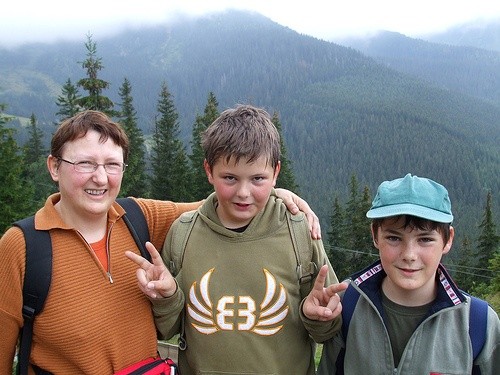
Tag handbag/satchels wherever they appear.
[112,356,177,374]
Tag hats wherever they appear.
[366,173,454,227]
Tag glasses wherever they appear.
[54,157,128,175]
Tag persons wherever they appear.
[313,172,500,375]
[0,106,323,375]
[122,104,348,375]
